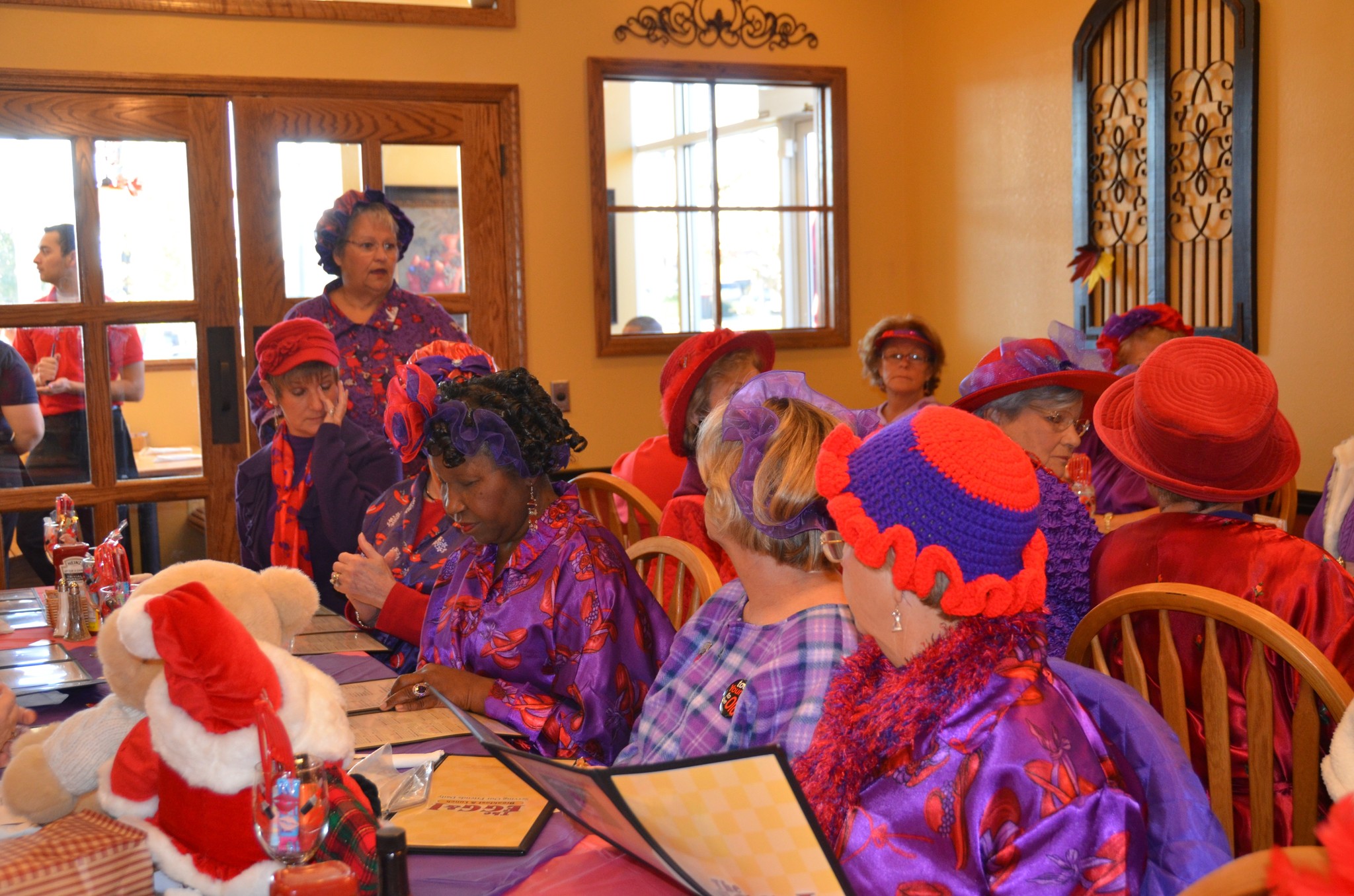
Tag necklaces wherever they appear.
[424,483,437,501]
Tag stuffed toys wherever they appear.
[0,559,320,829]
[96,581,357,896]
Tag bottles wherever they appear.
[374,830,417,896]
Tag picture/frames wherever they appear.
[384,183,619,324]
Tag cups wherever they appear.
[251,757,329,866]
[44,520,85,566]
[101,585,137,619]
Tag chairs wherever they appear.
[0,466,1354,896]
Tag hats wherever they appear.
[1093,338,1300,500]
[384,340,497,464]
[814,404,1049,619]
[875,330,933,351]
[255,317,339,380]
[118,582,307,795]
[660,328,775,458]
[1097,303,1194,371]
[315,183,415,275]
[950,321,1122,425]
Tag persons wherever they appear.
[246,189,471,442]
[0,679,38,771]
[950,320,1122,478]
[620,317,665,337]
[330,335,504,678]
[10,225,162,576]
[378,362,682,767]
[643,328,783,634]
[1,338,58,588]
[608,366,874,761]
[1085,334,1352,860]
[235,318,406,620]
[858,311,947,430]
[1073,299,1199,515]
[788,404,1145,896]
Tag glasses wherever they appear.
[1024,404,1090,438]
[877,352,932,364]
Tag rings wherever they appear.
[329,572,344,587]
[412,682,430,699]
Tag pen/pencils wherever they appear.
[43,343,55,387]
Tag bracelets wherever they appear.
[355,610,376,628]
